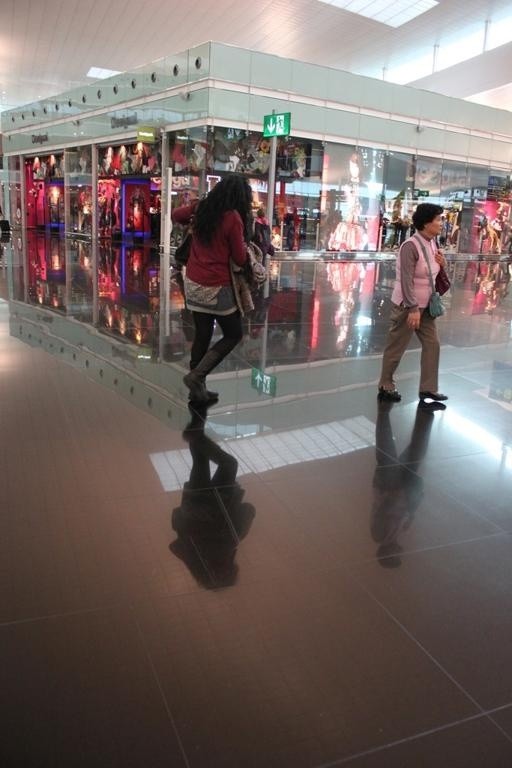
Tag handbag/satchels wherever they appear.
[435,240,450,295]
[175,230,193,266]
[428,291,445,317]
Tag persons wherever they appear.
[165,172,272,405]
[378,391,448,574]
[325,261,367,353]
[375,204,448,400]
[253,208,272,268]
[170,399,256,590]
[329,151,370,250]
[248,281,272,339]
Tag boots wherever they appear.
[184,350,223,402]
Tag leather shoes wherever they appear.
[378,384,402,399]
[419,390,447,402]
